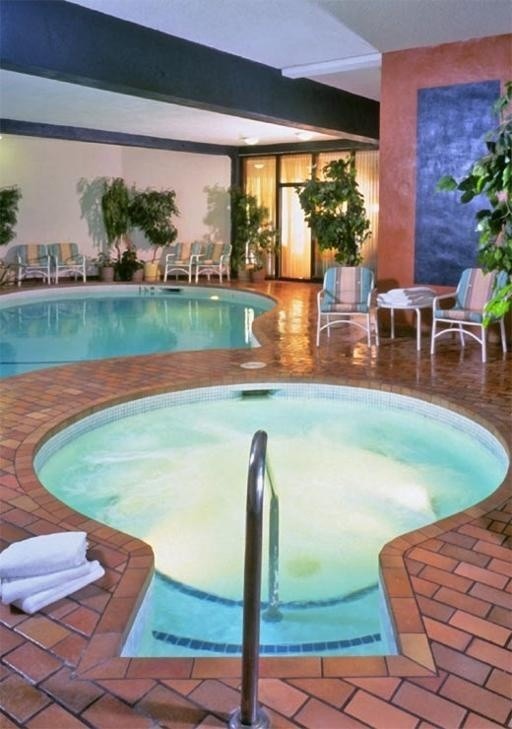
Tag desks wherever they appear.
[374,302,440,351]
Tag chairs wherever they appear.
[429,267,509,363]
[315,266,380,349]
[163,240,233,284]
[16,242,87,287]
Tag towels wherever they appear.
[376,288,436,307]
[0,530,105,613]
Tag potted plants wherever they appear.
[227,184,280,283]
[1,184,22,287]
[92,176,181,283]
